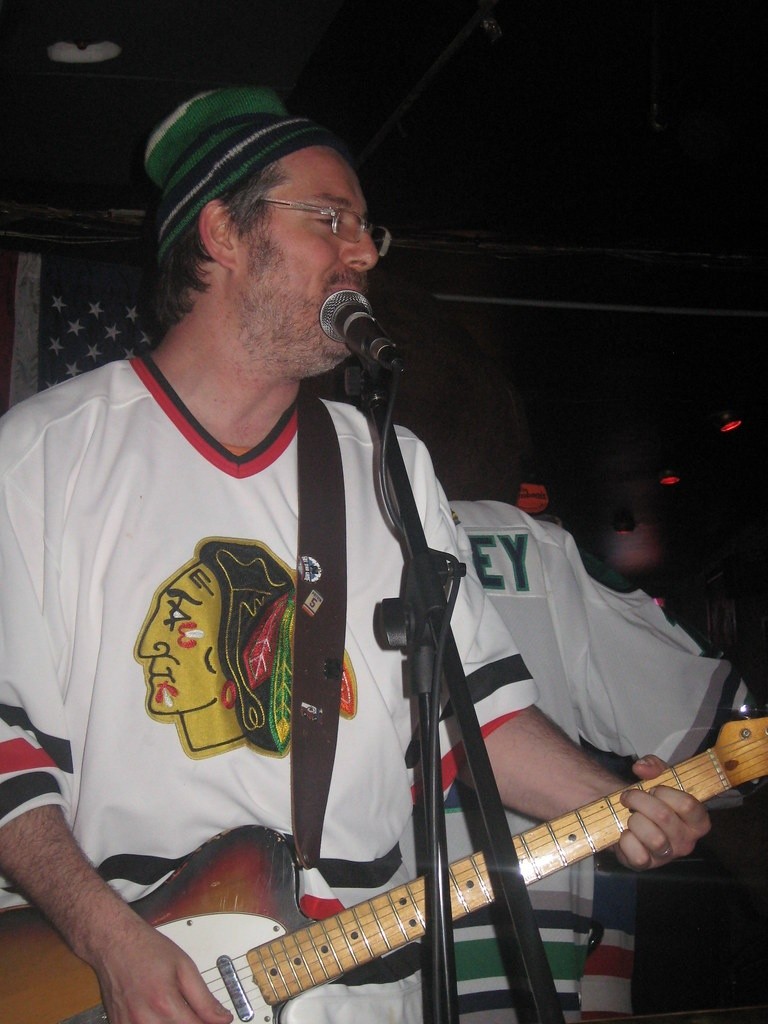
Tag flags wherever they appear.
[0,248,157,414]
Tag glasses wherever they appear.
[242,196,392,257]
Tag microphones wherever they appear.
[317,290,407,375]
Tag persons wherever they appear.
[0,85,713,1024]
[446,484,767,1024]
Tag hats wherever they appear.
[143,87,355,262]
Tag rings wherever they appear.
[658,847,671,857]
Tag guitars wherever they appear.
[0,713,768,1024]
